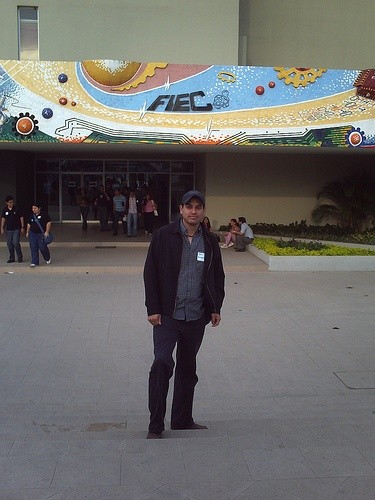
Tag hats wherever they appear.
[182,190,206,204]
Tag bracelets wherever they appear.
[234,231,235,234]
[21,227,24,228]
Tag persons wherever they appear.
[92,185,156,238]
[27,203,52,267]
[229,217,254,252]
[201,217,212,233]
[0,196,25,263]
[143,190,225,439]
[76,187,90,231]
[219,219,241,248]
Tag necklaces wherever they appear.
[187,235,194,238]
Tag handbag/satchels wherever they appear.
[43,233,54,244]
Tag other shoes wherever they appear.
[18,257,23,263]
[29,263,36,267]
[182,423,208,429]
[47,258,51,264]
[146,431,162,439]
[7,260,15,263]
[234,246,238,249]
[236,249,244,252]
